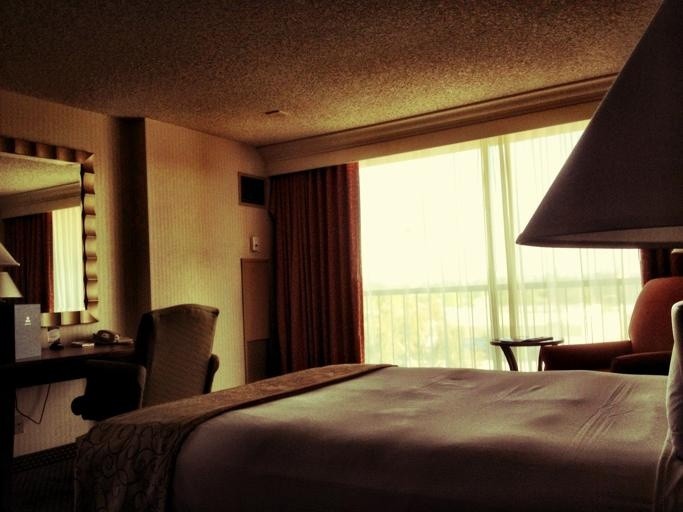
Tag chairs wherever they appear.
[545,274,682,372]
[75,304,219,415]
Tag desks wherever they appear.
[492,334,559,372]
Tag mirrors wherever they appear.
[0,135,99,327]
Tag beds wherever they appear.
[74,275,683,511]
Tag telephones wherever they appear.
[94,330,119,344]
